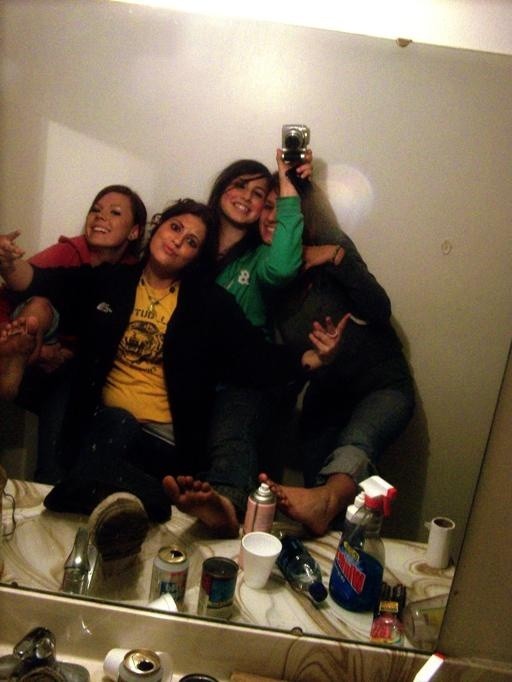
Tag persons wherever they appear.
[0,125,414,538]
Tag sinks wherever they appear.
[0,510,212,607]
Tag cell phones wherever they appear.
[286,165,315,202]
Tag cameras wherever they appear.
[282,124,310,161]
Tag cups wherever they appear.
[148,592,180,612]
[405,593,450,652]
[102,647,172,682]
[240,533,283,591]
[425,516,457,571]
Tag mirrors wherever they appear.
[0,0,506,651]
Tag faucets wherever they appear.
[57,526,104,598]
[0,623,89,682]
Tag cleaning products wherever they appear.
[328,476,398,609]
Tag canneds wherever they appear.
[198,556,238,619]
[117,648,163,682]
[179,673,220,681]
[149,545,189,608]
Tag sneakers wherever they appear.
[87,492,150,561]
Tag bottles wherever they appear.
[342,490,374,550]
[372,601,405,652]
[278,531,328,604]
